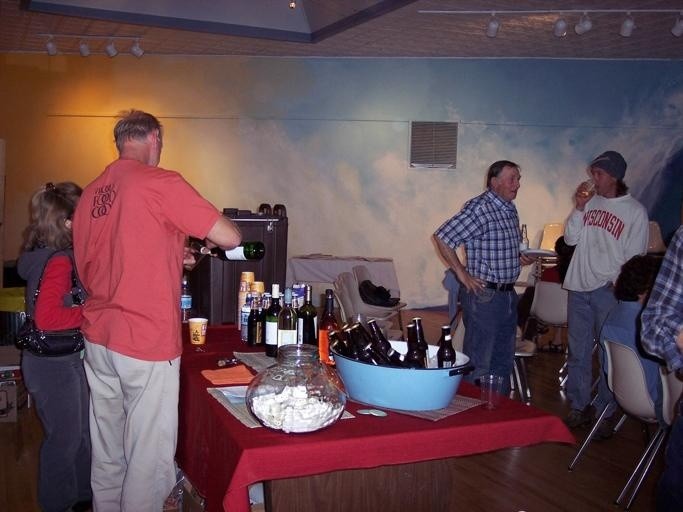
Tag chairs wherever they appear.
[333,264,407,341]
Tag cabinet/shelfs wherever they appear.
[185,204,288,330]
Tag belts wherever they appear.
[484,281,516,292]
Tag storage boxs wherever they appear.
[0,376,28,423]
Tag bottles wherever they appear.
[327,316,457,370]
[190,237,207,254]
[180,275,192,325]
[241,281,335,366]
[519,224,529,253]
[209,240,266,263]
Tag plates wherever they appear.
[520,247,557,256]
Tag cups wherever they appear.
[183,246,206,271]
[479,375,504,410]
[238,271,265,331]
[576,178,596,197]
[187,318,207,345]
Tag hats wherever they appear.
[593,151,627,180]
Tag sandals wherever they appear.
[536,341,566,354]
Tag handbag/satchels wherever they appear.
[360,280,400,307]
[15,298,85,358]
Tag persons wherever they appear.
[72,109,242,512]
[16,181,92,511]
[639,224,683,481]
[599,253,664,428]
[433,160,538,396]
[561,151,650,436]
[518,236,576,326]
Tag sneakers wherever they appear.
[565,410,593,428]
[536,323,549,335]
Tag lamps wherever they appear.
[485,8,683,39]
[46,31,145,59]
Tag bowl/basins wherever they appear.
[330,340,471,411]
